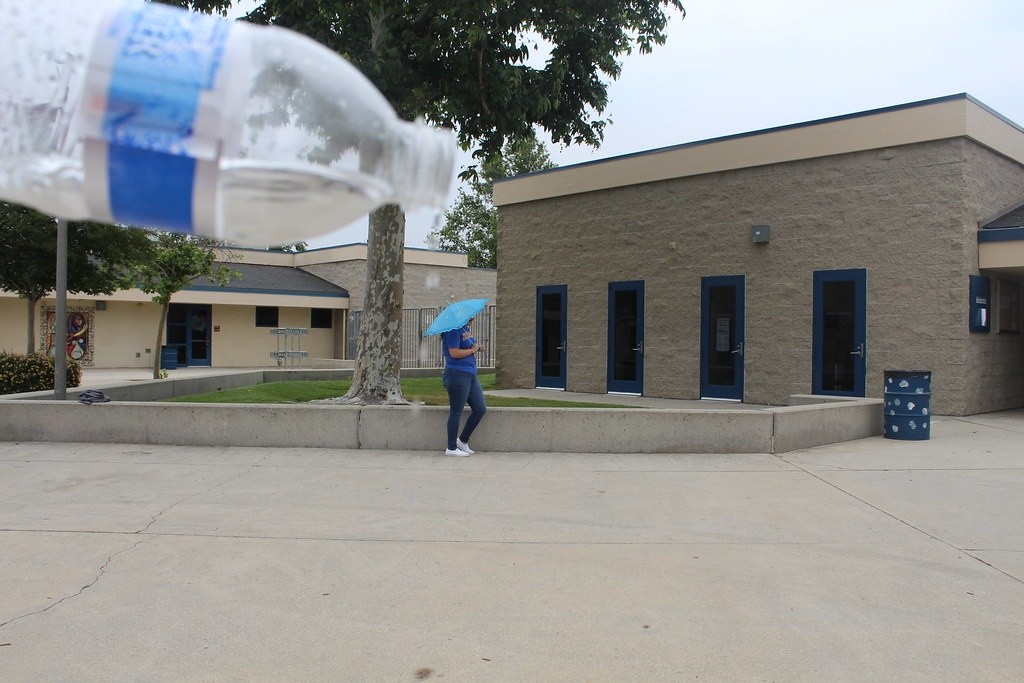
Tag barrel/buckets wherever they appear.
[882,369,933,441]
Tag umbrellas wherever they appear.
[424,298,490,345]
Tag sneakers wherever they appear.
[456,437,475,454]
[445,447,469,457]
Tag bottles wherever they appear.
[0,0,457,247]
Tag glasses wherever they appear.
[469,320,472,322]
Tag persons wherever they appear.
[440,317,486,456]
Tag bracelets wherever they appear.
[471,349,476,353]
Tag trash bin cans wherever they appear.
[161,345,179,370]
[883,369,931,441]
